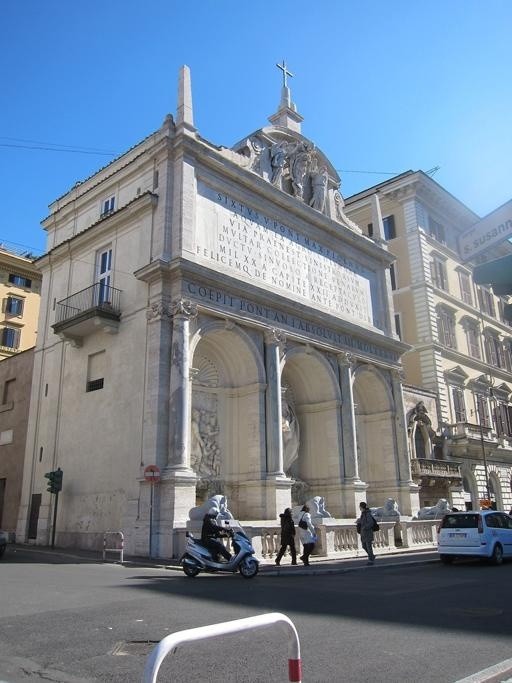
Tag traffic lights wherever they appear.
[44,470,63,492]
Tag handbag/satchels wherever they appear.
[298,520,307,530]
[357,516,380,534]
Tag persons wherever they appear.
[358,501,376,560]
[202,506,232,561]
[298,504,318,565]
[312,164,327,213]
[274,508,298,565]
[270,141,289,189]
[292,152,308,197]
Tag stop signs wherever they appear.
[145,464,160,484]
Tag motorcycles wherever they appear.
[178,521,259,579]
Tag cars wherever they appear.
[438,510,512,564]
